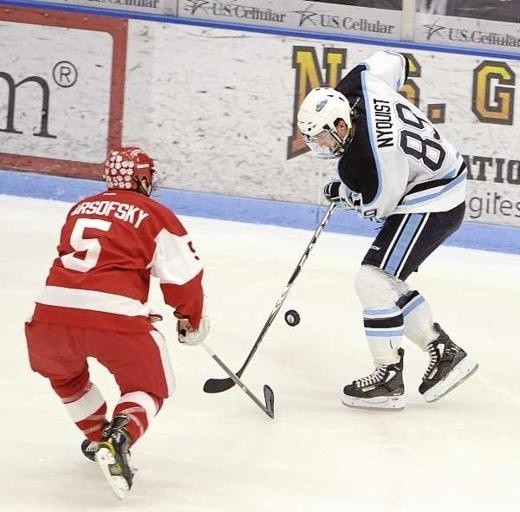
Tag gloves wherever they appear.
[323,182,352,212]
[176,318,210,346]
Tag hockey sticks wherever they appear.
[201,201,337,391]
[196,339,275,419]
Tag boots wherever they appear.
[81,415,133,490]
[418,323,467,395]
[343,347,405,398]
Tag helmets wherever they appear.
[296,86,354,159]
[105,146,156,197]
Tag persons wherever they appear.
[297,48,467,398]
[25,146,203,499]
